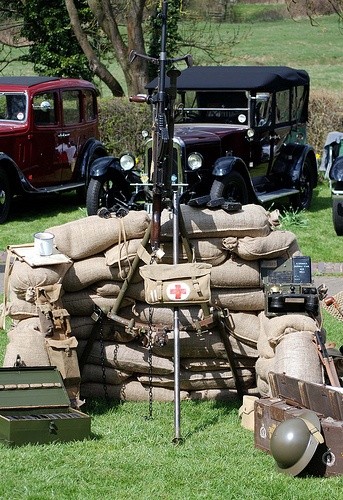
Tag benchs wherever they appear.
[34,108,80,125]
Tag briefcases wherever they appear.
[0,366,91,450]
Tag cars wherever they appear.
[0,75,110,224]
[86,65,318,219]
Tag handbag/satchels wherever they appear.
[139,263,212,304]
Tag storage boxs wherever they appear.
[254,371,343,478]
[0,366,92,444]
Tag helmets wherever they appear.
[270,411,324,476]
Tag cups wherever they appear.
[34,232,54,256]
